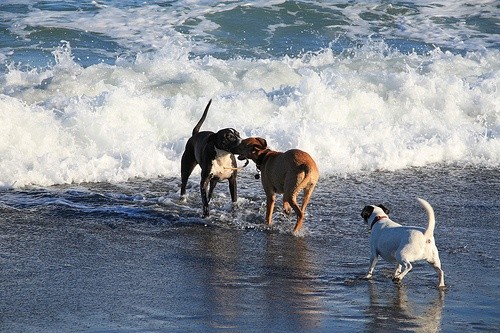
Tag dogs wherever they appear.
[359,197,445,288]
[178,98,244,221]
[231,137,319,234]
